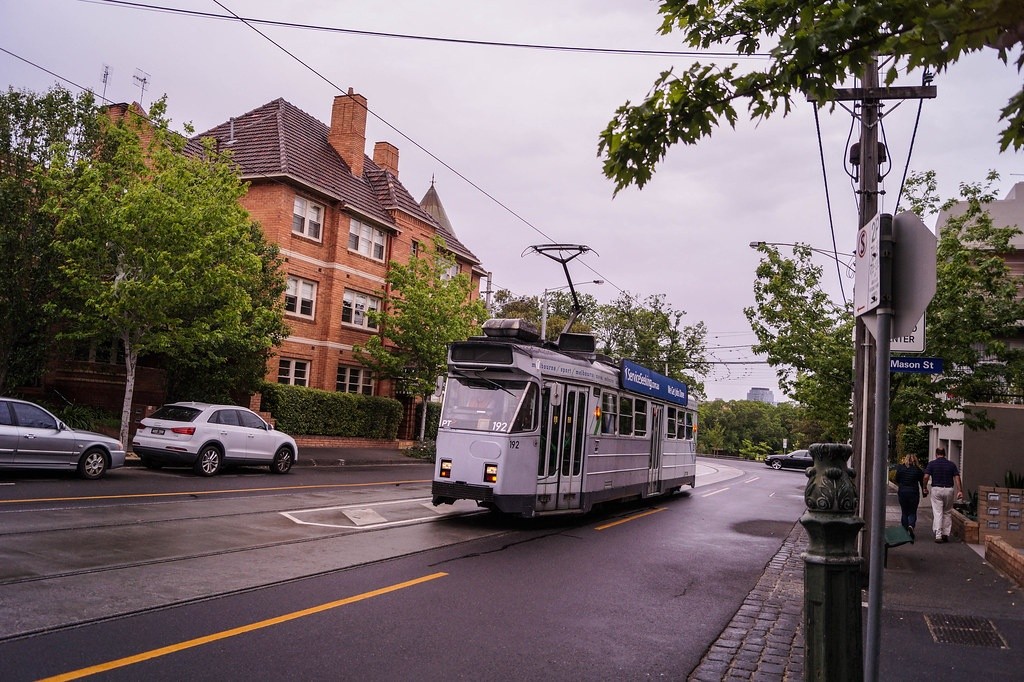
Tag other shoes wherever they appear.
[908,526,916,540]
[934,540,943,544]
[942,535,948,542]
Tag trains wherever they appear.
[431,319,698,528]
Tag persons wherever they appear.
[923,448,963,543]
[895,454,927,540]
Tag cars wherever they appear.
[764,450,814,470]
[0,398,126,480]
[132,402,298,477]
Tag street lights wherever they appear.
[749,242,856,469]
[541,280,604,340]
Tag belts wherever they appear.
[932,485,952,487]
